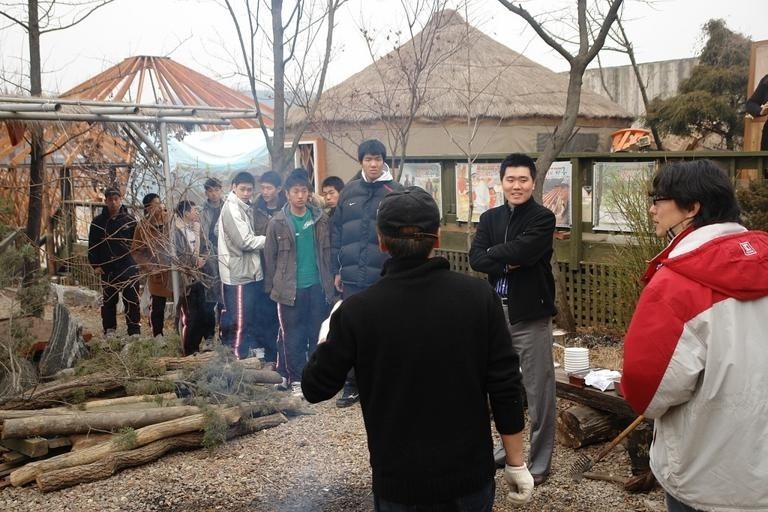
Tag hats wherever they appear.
[376,187,440,239]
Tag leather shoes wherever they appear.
[531,473,547,486]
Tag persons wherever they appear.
[329,139,404,408]
[469,153,559,486]
[746,73,768,183]
[300,186,534,512]
[90,188,142,337]
[621,159,768,512]
[130,168,344,397]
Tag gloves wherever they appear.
[503,462,535,508]
[315,299,343,346]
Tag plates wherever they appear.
[563,347,590,375]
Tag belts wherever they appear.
[500,298,508,308]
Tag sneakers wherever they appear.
[274,376,304,399]
[336,384,359,408]
[202,338,217,353]
[155,334,169,349]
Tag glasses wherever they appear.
[651,196,673,206]
